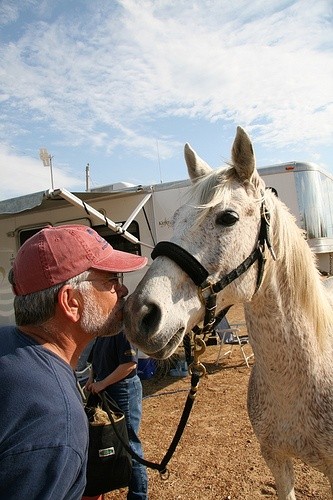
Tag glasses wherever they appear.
[56,273,124,302]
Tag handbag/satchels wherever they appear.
[83,383,133,496]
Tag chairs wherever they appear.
[214,315,255,368]
[73,353,97,400]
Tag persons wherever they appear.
[85,328,148,500]
[0,223,148,500]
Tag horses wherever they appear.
[120,124,333,500]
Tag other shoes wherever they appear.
[81,493,104,500]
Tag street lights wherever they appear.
[39,147,54,190]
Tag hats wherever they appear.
[8,224,148,297]
